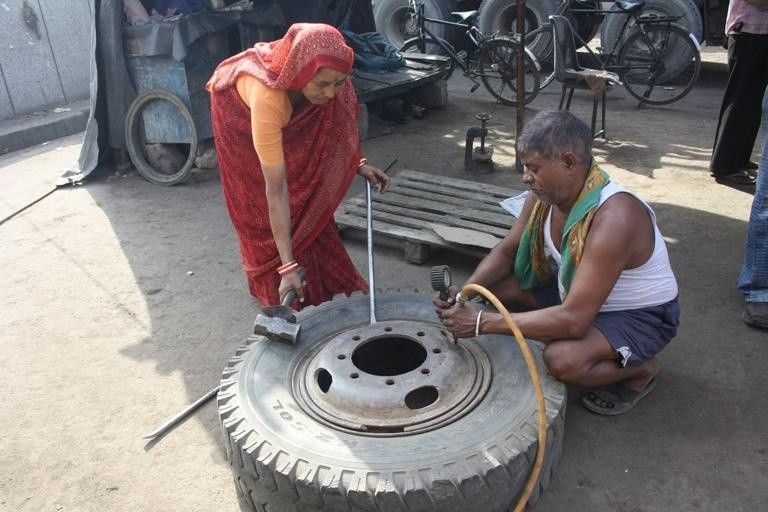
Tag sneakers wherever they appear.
[741,302,768,328]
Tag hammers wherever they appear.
[252,266,306,345]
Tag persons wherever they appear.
[429,106,683,420]
[704,0,767,189]
[735,90,767,330]
[195,21,389,313]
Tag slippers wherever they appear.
[582,377,656,415]
[710,160,758,185]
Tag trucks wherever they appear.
[368,1,730,87]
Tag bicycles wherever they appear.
[502,0,706,111]
[391,1,544,111]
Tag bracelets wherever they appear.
[276,258,302,276]
[356,157,368,168]
[474,309,484,339]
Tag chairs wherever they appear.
[548,15,622,147]
